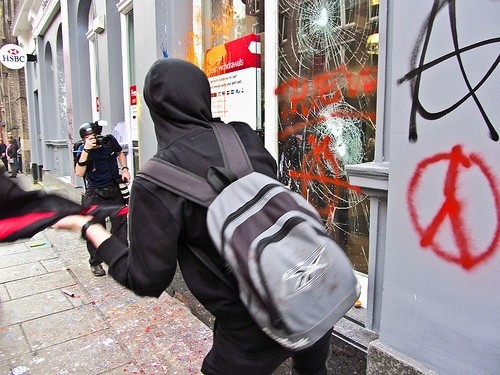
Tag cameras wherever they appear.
[92,120,111,147]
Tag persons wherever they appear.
[72,121,131,276]
[0,138,23,178]
[51,57,333,375]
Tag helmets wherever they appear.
[79,122,98,139]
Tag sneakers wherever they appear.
[90,263,106,277]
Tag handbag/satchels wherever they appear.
[8,158,14,164]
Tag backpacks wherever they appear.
[133,121,361,352]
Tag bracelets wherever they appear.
[81,218,101,239]
[83,149,91,153]
[122,167,128,170]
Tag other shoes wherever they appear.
[8,175,16,178]
